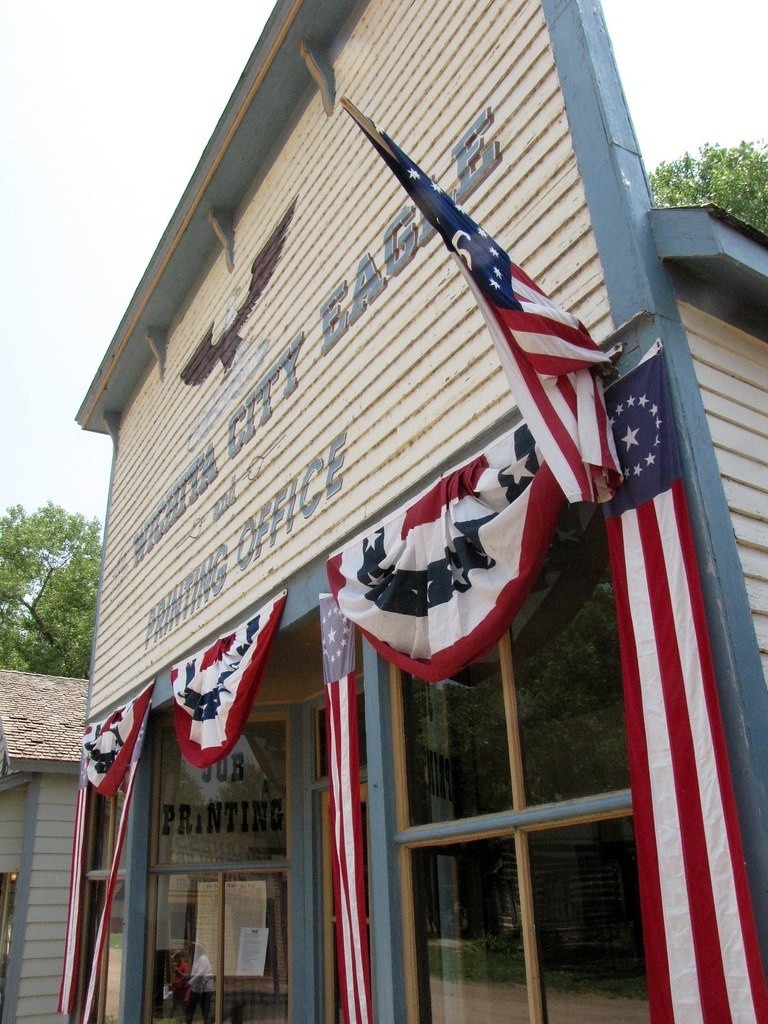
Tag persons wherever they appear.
[166,948,191,1018]
[184,939,213,1024]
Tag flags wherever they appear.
[349,106,624,504]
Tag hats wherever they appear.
[170,950,182,959]
